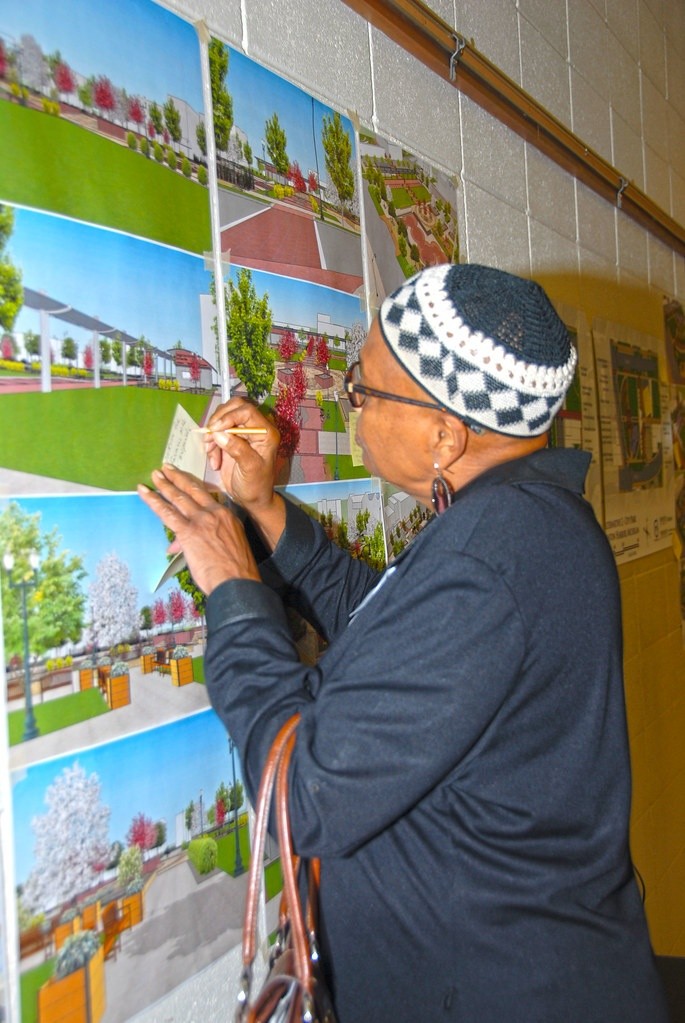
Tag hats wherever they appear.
[376,262,579,439]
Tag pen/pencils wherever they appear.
[197,427,267,435]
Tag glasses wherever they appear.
[343,359,488,436]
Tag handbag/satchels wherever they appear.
[229,712,337,1023]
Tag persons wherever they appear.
[137,262,665,1023]
